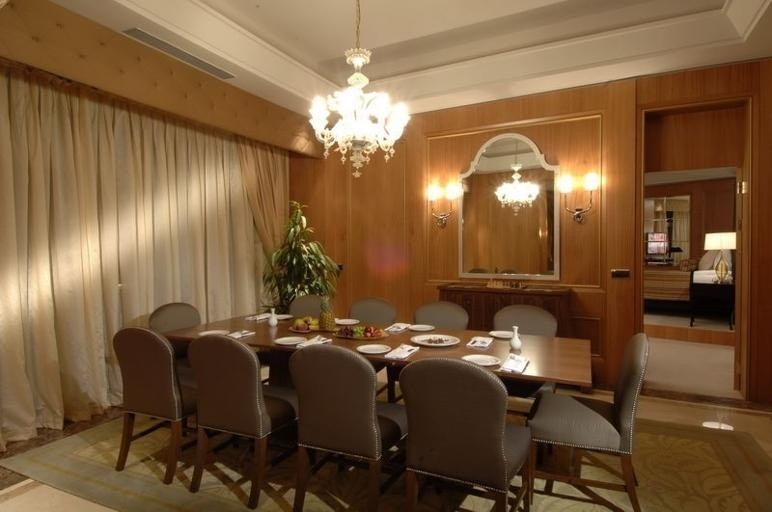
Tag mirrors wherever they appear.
[455,133,562,283]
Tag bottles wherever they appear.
[268,307,278,327]
[509,326,521,349]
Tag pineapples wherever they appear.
[318,295,335,332]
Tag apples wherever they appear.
[292,319,304,331]
[303,315,312,323]
[308,318,319,325]
[309,324,320,330]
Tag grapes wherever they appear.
[336,324,354,339]
[353,326,364,338]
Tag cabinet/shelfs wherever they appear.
[687,270,735,330]
[435,283,572,336]
[642,265,693,316]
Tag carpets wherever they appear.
[0,381,772,512]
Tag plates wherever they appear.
[462,353,500,366]
[271,318,361,346]
[356,344,391,354]
[410,324,434,332]
[198,314,294,337]
[489,330,520,338]
[409,334,460,347]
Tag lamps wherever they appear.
[492,140,542,217]
[558,172,601,224]
[423,181,464,230]
[704,231,737,285]
[307,1,412,178]
